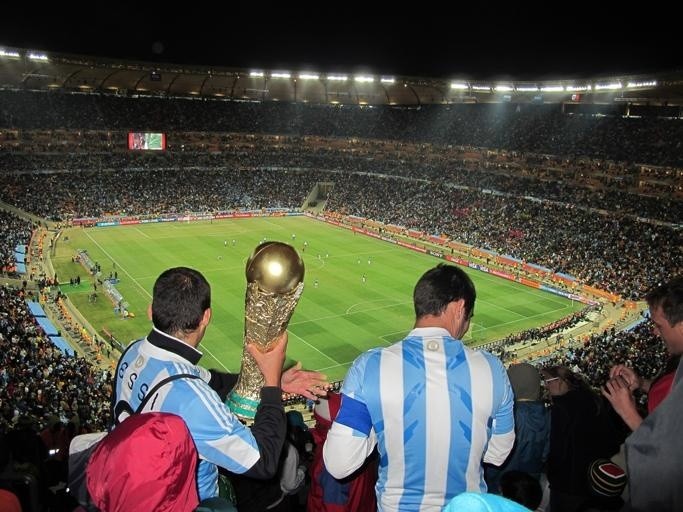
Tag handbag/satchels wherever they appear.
[69,431,112,500]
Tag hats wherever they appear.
[286,410,308,432]
[314,393,342,426]
[442,492,535,512]
[586,459,627,497]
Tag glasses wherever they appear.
[545,377,561,385]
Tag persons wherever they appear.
[484,277,681,512]
[112,267,334,511]
[447,87,682,278]
[292,234,295,239]
[260,237,266,245]
[112,88,307,221]
[358,256,376,263]
[2,88,112,512]
[304,241,307,246]
[321,264,536,512]
[306,87,448,256]
[225,239,236,246]
[318,253,330,258]
